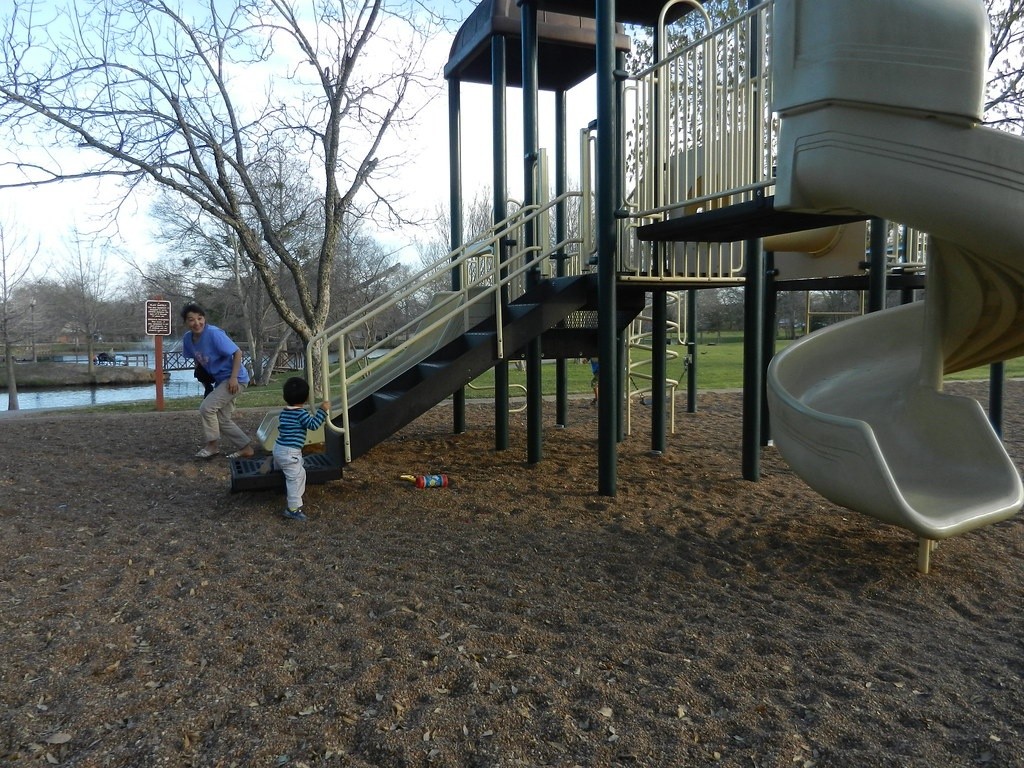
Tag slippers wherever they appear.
[196,447,220,458]
[226,452,251,459]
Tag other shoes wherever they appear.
[282,509,308,522]
[260,456,274,475]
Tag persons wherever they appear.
[258,377,330,520]
[590,358,599,405]
[181,303,256,457]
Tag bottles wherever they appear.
[416,475,456,488]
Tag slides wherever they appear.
[255,284,495,453]
[776,105,1024,541]
